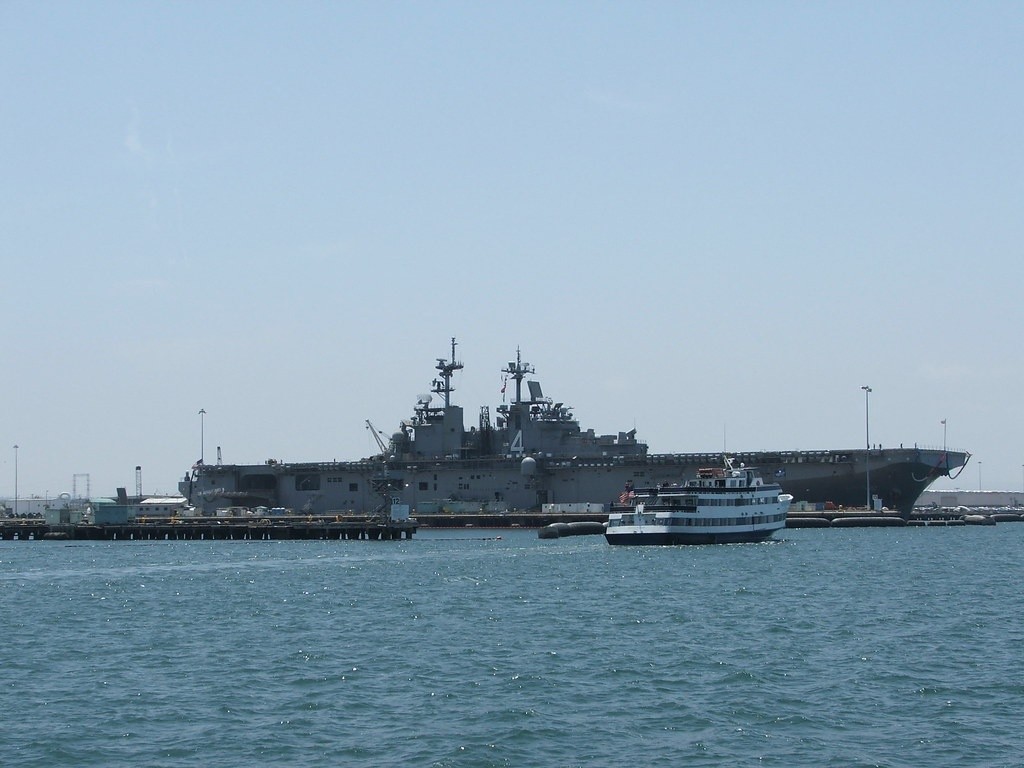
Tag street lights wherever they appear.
[13,444,19,520]
[197,408,207,517]
[978,461,983,490]
[861,384,872,511]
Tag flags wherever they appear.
[619,491,629,503]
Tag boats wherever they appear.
[178,336,971,515]
[603,462,795,546]
